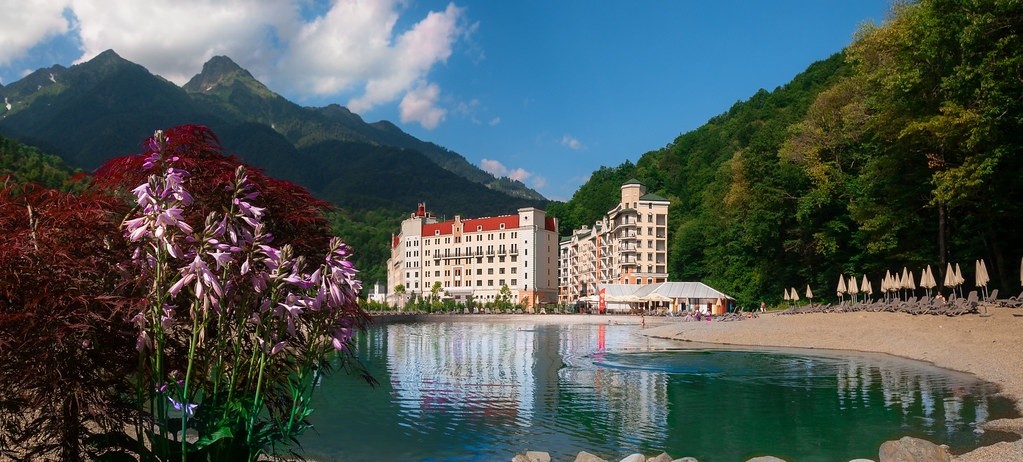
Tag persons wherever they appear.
[935,290,945,304]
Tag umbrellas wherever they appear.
[1020,257,1023,289]
[783,284,813,309]
[880,266,915,305]
[956,262,965,298]
[943,262,958,301]
[926,264,937,305]
[837,273,873,305]
[975,259,990,303]
[919,268,929,297]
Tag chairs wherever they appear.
[473,307,479,314]
[639,288,1023,323]
[528,307,536,315]
[464,308,470,315]
[363,308,461,316]
[485,307,492,314]
[554,308,562,314]
[540,307,547,315]
[506,309,512,314]
[494,308,502,314]
[515,309,524,314]
[563,309,572,314]
